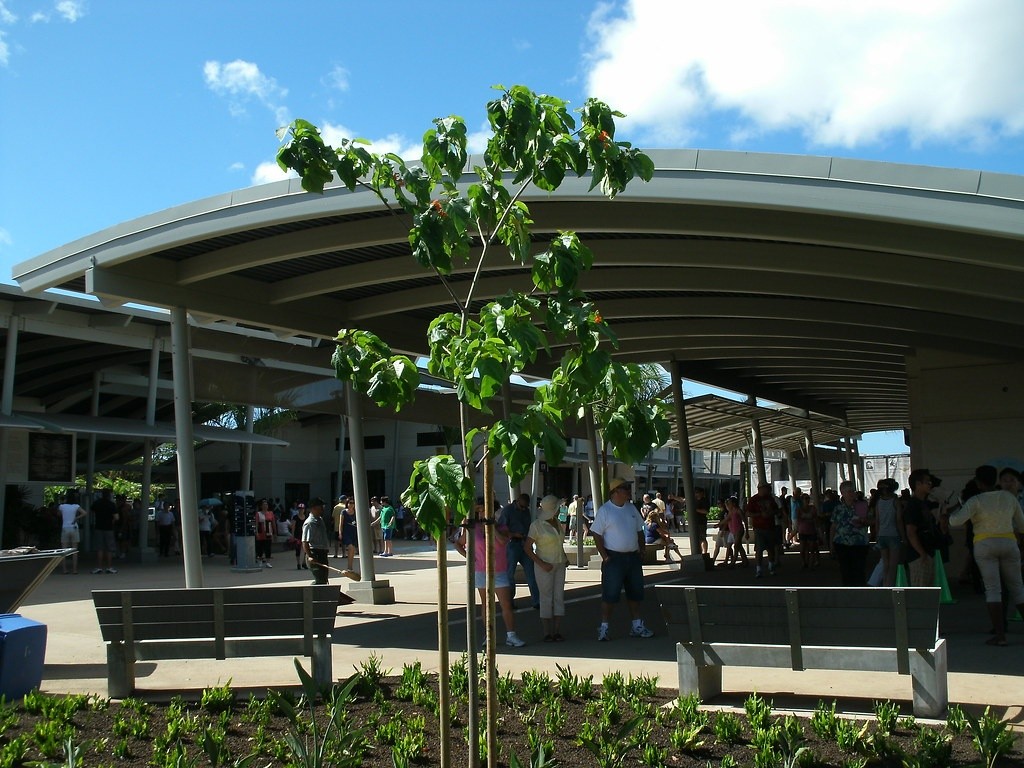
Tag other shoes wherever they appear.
[769,567,776,574]
[106,567,117,573]
[297,564,302,570]
[754,572,761,578]
[265,563,273,568]
[629,620,655,637]
[543,633,564,642]
[258,559,262,568]
[984,636,1008,646]
[597,624,610,641]
[301,563,308,570]
[91,568,103,574]
[379,552,393,557]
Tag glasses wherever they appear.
[518,502,530,509]
[71,522,77,529]
[348,502,355,505]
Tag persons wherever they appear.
[522,496,569,641]
[589,477,657,640]
[558,467,1024,601]
[949,465,1024,645]
[456,497,526,647]
[497,494,541,612]
[43,488,457,586]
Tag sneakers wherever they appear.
[482,635,487,645]
[506,635,525,647]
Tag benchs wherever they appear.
[641,543,664,565]
[655,582,947,719]
[91,584,341,701]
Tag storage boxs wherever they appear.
[0,613,48,703]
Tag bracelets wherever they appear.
[512,532,515,537]
[603,556,608,562]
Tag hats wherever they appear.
[340,495,347,500]
[306,495,328,508]
[757,480,771,489]
[298,503,305,509]
[536,495,567,521]
[608,475,635,492]
